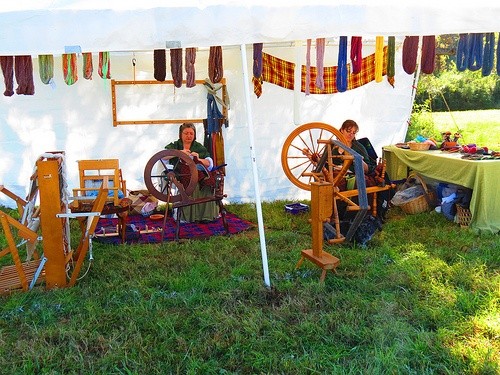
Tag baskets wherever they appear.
[409,141,431,151]
[456,203,472,228]
[398,173,434,214]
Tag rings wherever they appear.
[196,153,198,156]
[196,156,198,157]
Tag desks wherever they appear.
[382,145,500,236]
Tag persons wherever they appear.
[323,120,383,249]
[161,122,220,224]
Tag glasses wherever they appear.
[344,128,357,135]
